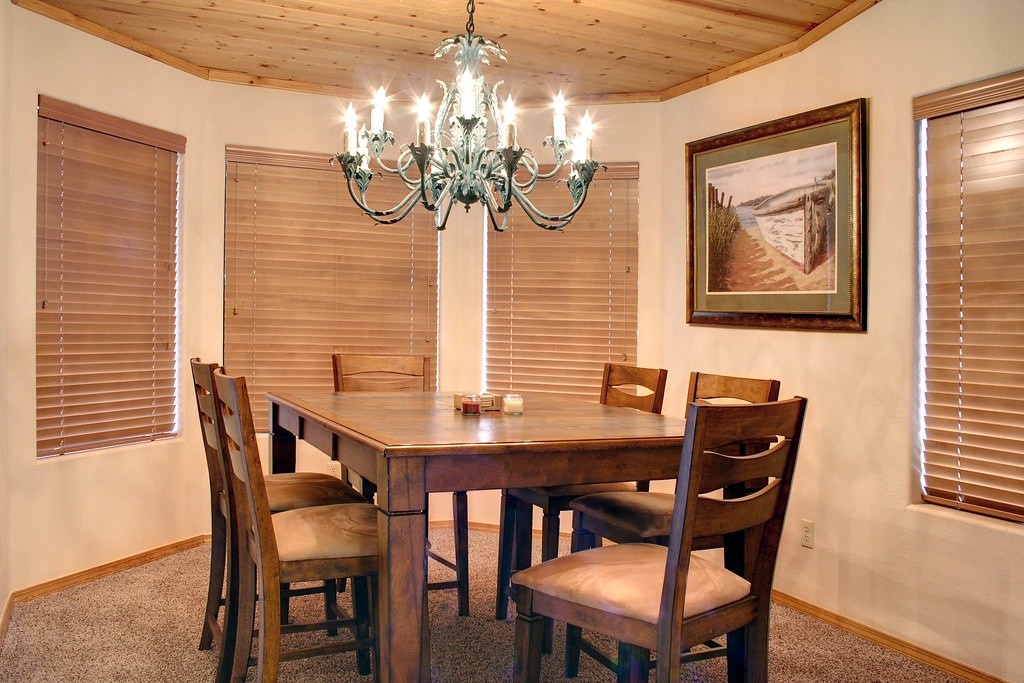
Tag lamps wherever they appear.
[331,2,600,230]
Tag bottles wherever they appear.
[503,394,523,415]
[461,393,482,416]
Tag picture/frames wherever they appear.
[680,97,871,332]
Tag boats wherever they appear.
[752,181,832,275]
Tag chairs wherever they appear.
[493,360,666,655]
[328,350,473,619]
[538,368,782,678]
[181,356,355,657]
[206,363,380,683]
[511,394,808,683]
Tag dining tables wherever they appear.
[258,384,739,682]
